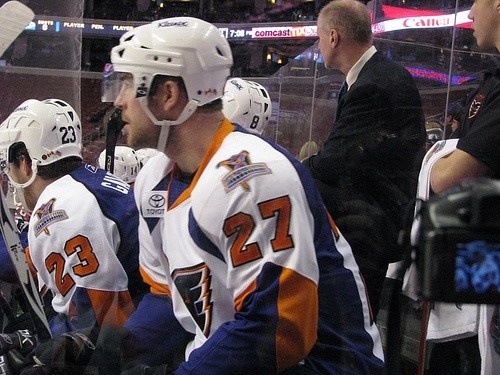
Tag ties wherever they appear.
[331,79,348,127]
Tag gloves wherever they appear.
[20,327,137,375]
[0,329,38,375]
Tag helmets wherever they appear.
[111,17,233,106]
[98,145,143,183]
[0,98,83,166]
[220,77,273,135]
[136,148,161,166]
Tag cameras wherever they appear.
[416,176,500,305]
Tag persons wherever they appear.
[16,17,386,375]
[221,77,272,136]
[417,0,500,375]
[96,145,161,184]
[0,169,46,337]
[297,0,427,320]
[0,99,190,375]
